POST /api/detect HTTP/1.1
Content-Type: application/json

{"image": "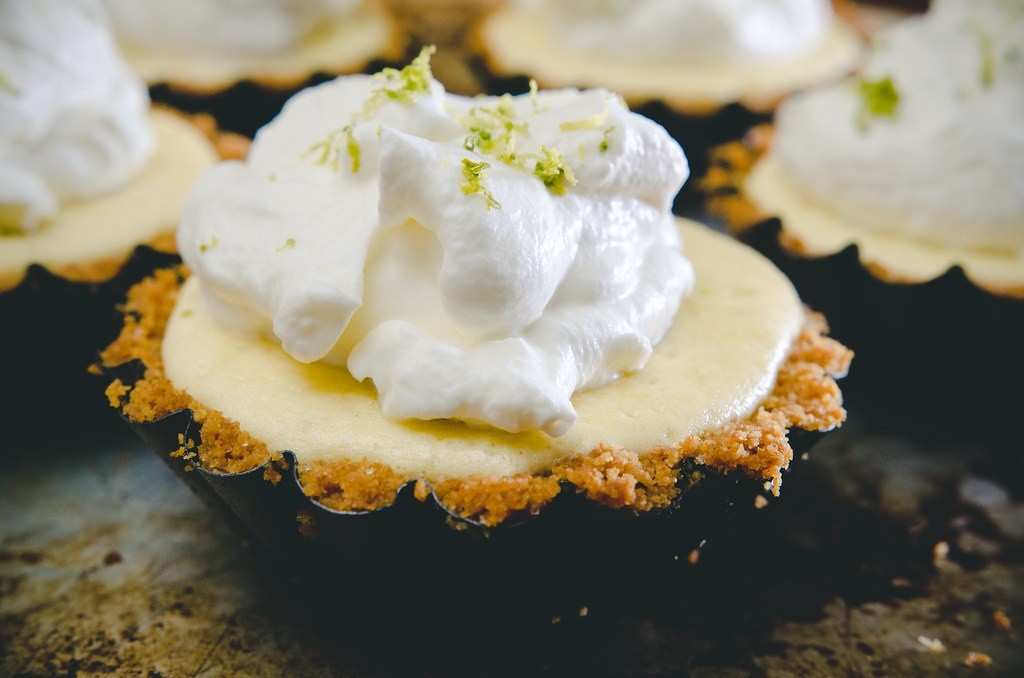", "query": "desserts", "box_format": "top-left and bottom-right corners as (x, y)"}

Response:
top-left (0, 0), bottom-right (1024, 526)
